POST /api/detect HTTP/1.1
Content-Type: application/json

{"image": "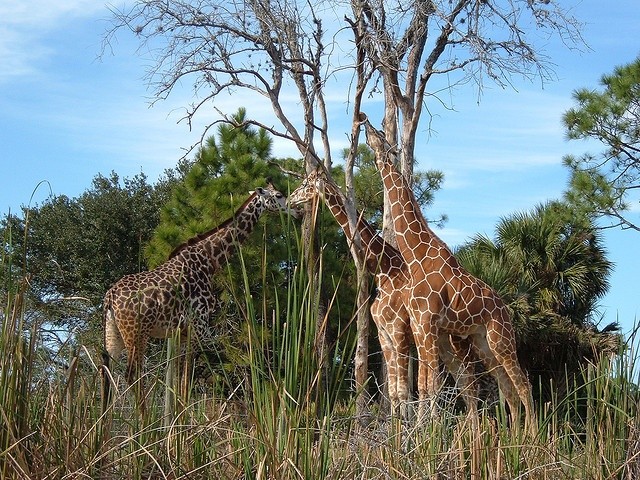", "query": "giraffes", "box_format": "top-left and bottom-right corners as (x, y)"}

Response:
top-left (285, 159), bottom-right (483, 419)
top-left (356, 112), bottom-right (539, 441)
top-left (101, 182), bottom-right (306, 421)
